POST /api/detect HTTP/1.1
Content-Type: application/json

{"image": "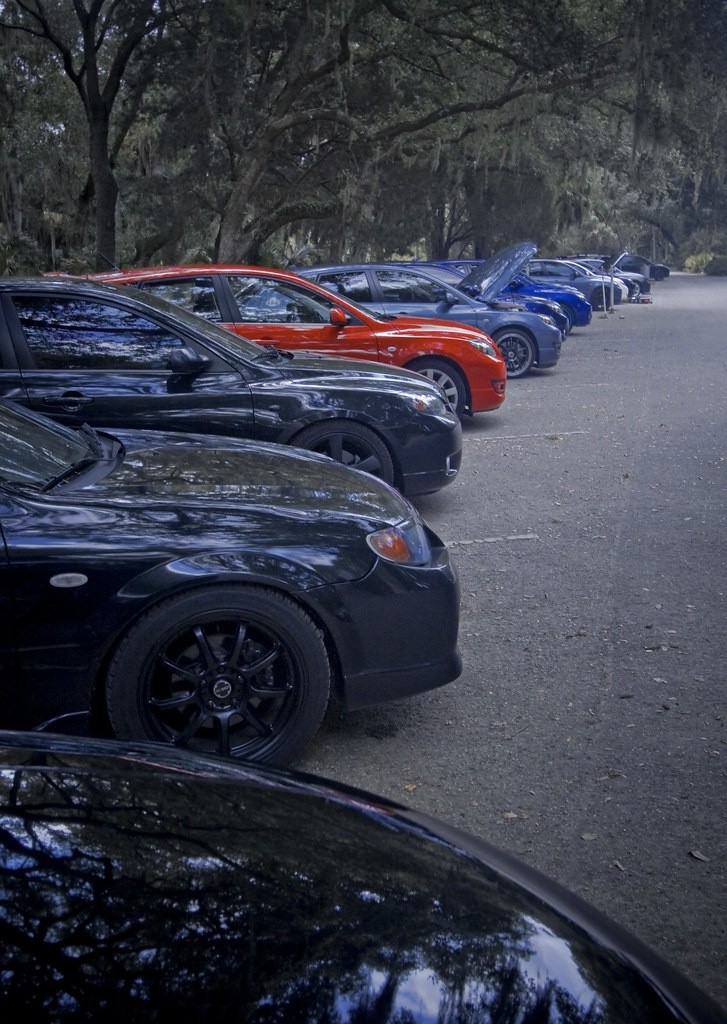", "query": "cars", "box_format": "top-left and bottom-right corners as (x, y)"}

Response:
top-left (1, 393), bottom-right (465, 776)
top-left (0, 282), bottom-right (466, 497)
top-left (345, 249), bottom-right (672, 340)
top-left (236, 246), bottom-right (567, 378)
top-left (44, 265), bottom-right (509, 423)
top-left (0, 726), bottom-right (727, 1023)
